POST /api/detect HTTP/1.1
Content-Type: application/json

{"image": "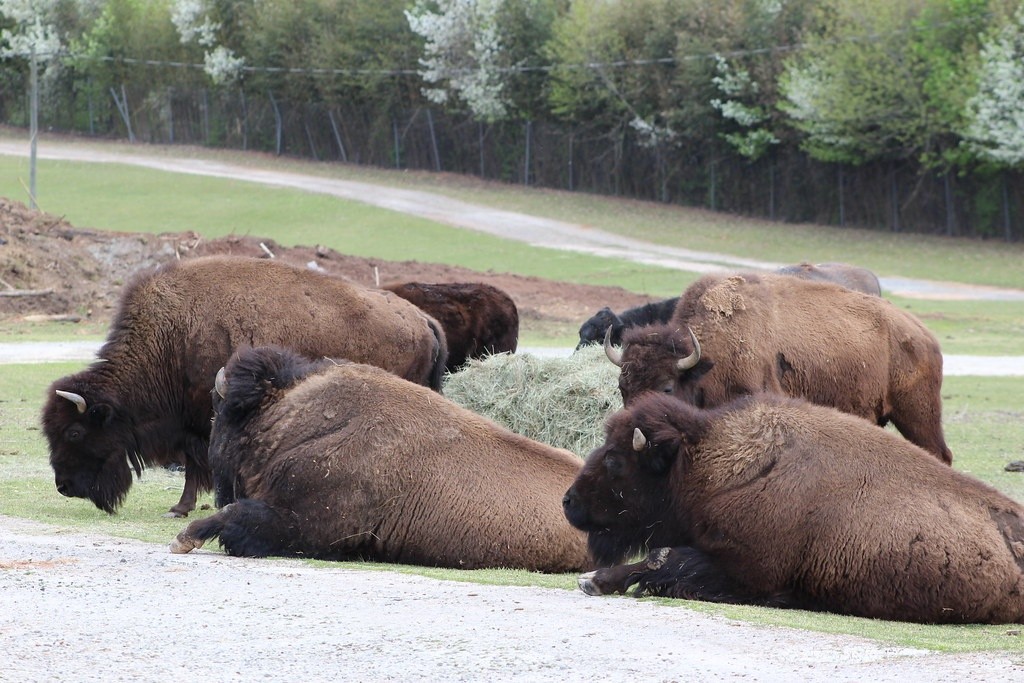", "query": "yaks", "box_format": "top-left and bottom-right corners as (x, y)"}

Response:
top-left (39, 231), bottom-right (1024, 626)
